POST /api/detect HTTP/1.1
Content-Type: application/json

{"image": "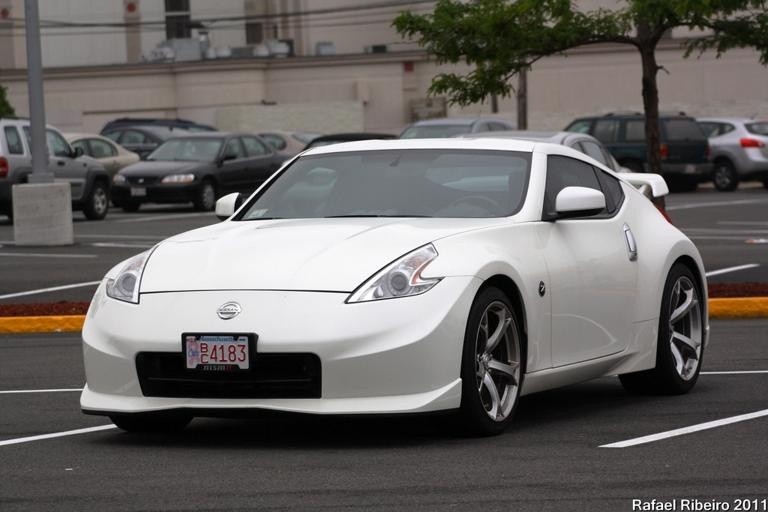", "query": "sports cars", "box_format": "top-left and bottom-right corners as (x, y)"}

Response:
top-left (72, 133), bottom-right (718, 447)
